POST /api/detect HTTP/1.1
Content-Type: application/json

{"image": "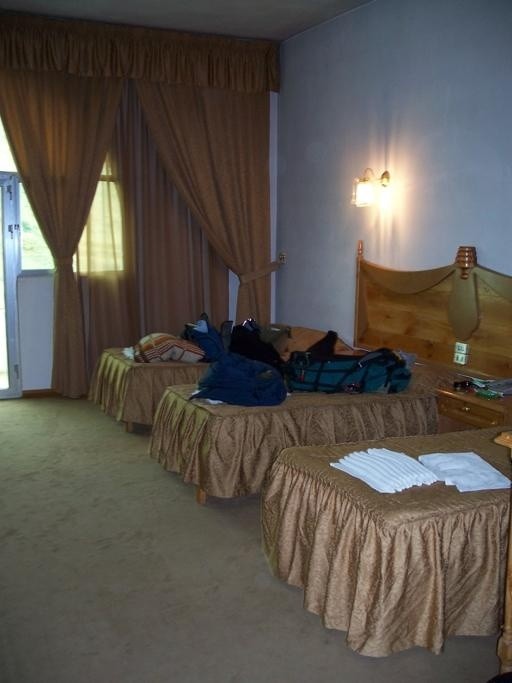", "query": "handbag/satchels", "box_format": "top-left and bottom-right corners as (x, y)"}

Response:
top-left (198, 351), bottom-right (286, 405)
top-left (287, 346), bottom-right (410, 393)
top-left (229, 324), bottom-right (284, 371)
top-left (180, 312), bottom-right (223, 363)
